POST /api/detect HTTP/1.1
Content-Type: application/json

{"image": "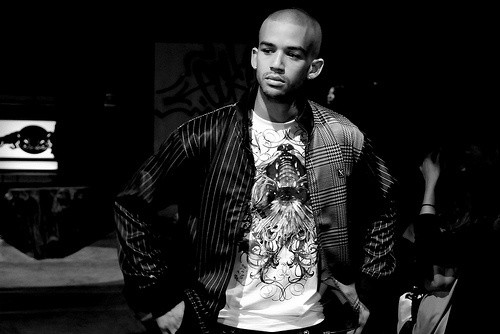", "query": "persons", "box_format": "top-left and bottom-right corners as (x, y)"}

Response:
top-left (116, 7), bottom-right (398, 334)
top-left (326, 79), bottom-right (500, 334)
